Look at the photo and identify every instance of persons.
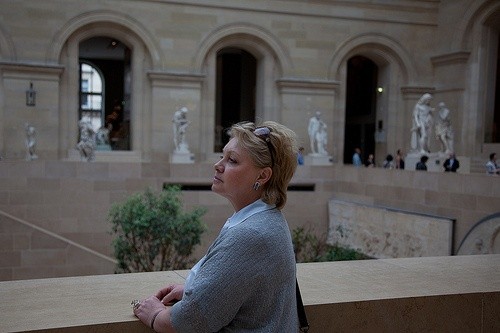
[296,143,500,180]
[1,88,460,173]
[133,119,302,333]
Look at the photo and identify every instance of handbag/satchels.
[296,277,309,331]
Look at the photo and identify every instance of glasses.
[253,127,276,170]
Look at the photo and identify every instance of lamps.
[378,86,383,95]
[25,82,36,106]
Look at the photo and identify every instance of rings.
[131,299,142,310]
